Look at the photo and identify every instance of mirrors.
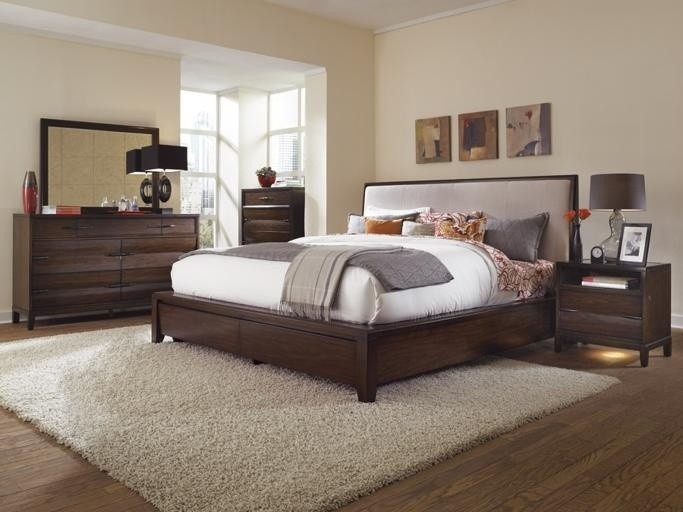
[39,116,158,213]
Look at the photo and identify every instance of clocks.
[591,246,603,262]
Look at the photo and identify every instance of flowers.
[564,206,589,223]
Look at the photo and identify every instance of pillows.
[344,205,551,264]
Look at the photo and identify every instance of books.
[41,204,82,215]
[581,276,640,291]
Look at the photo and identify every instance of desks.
[12,210,199,329]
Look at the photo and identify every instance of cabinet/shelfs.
[238,187,303,247]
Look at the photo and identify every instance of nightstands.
[554,257,673,366]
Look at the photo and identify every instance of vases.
[571,223,582,259]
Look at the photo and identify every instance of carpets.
[0,321,622,510]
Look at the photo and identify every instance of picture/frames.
[616,221,652,267]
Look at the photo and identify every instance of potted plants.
[255,165,276,188]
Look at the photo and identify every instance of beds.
[146,173,580,407]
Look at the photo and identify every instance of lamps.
[122,148,152,204]
[589,170,645,261]
[142,143,188,213]
